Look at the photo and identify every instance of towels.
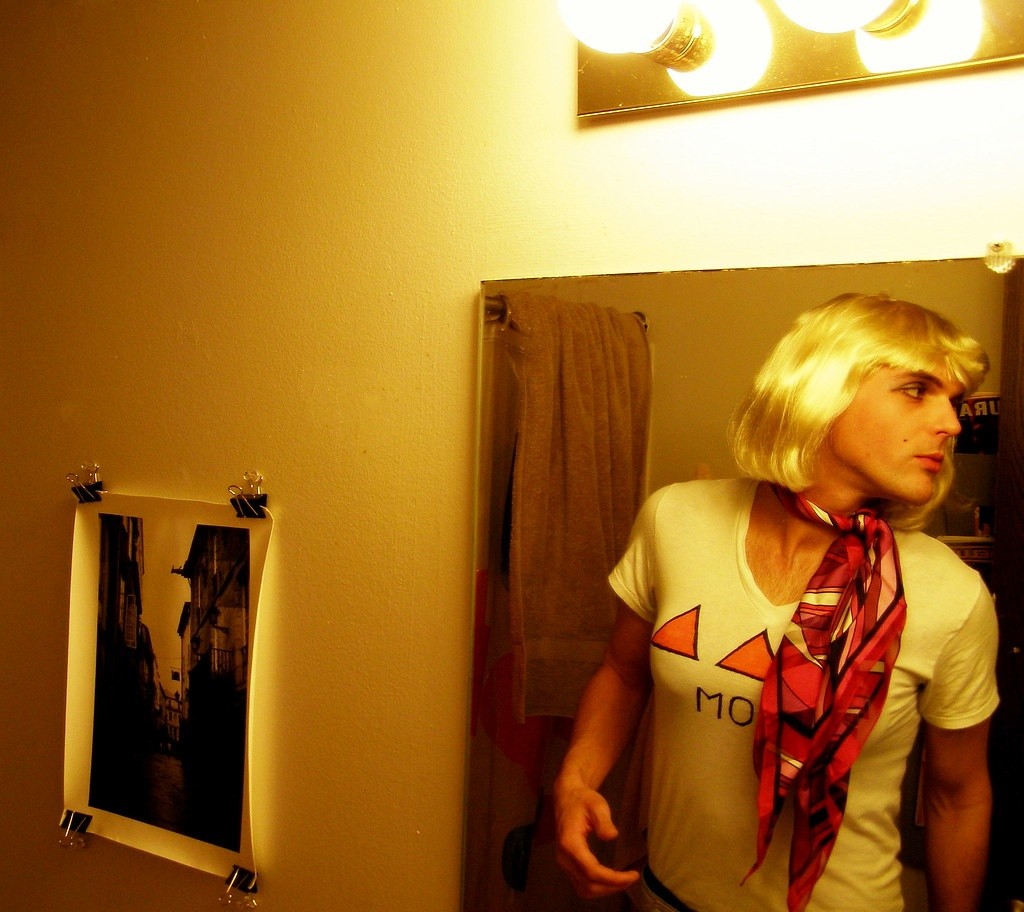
[486,289,652,726]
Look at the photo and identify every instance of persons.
[553,292,1000,912]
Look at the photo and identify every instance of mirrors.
[466,255,1024,912]
